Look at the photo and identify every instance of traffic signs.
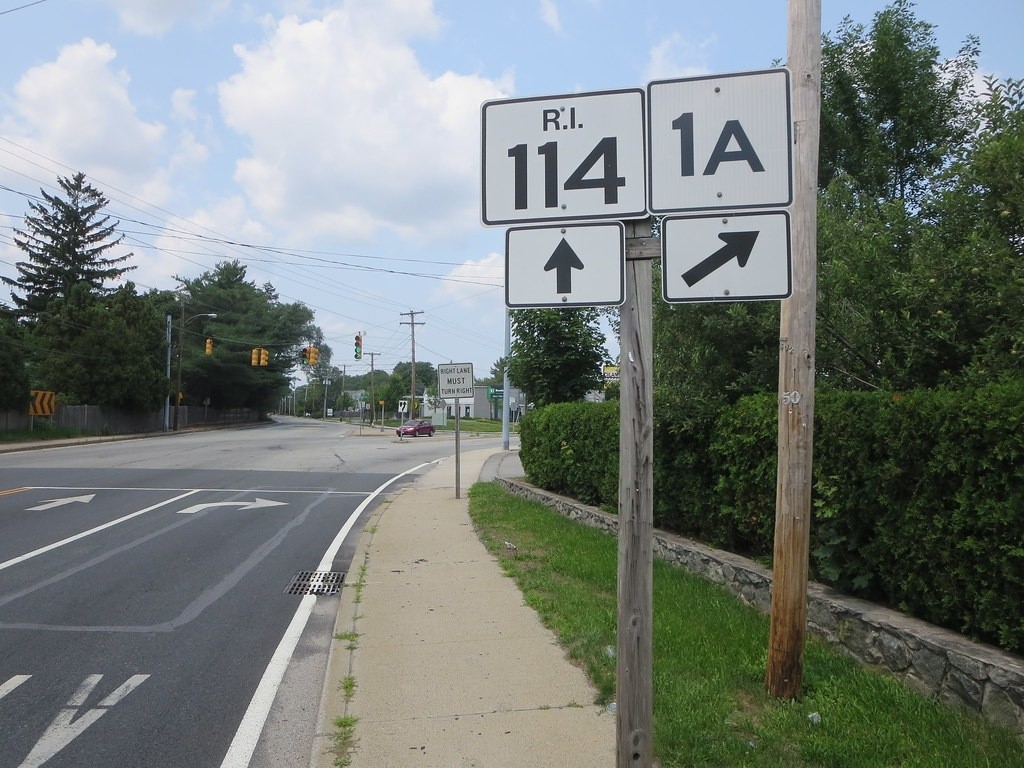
[30,390,54,415]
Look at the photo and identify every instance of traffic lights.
[260,349,268,366]
[355,335,362,359]
[310,347,318,364]
[302,348,310,365]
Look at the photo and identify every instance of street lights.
[173,314,217,431]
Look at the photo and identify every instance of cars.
[396,419,436,437]
